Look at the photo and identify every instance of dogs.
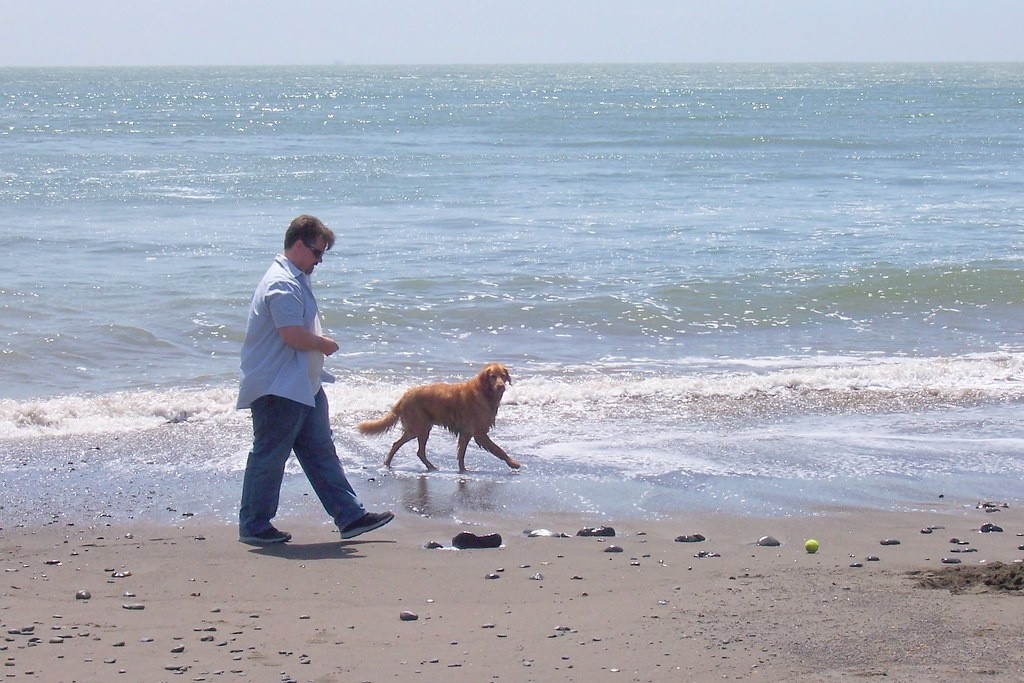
[358,363,520,471]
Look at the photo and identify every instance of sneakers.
[340,510,394,539]
[239,525,292,544]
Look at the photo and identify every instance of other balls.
[804,539,821,554]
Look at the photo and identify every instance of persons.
[235,215,395,544]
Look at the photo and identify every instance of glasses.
[308,245,323,258]
